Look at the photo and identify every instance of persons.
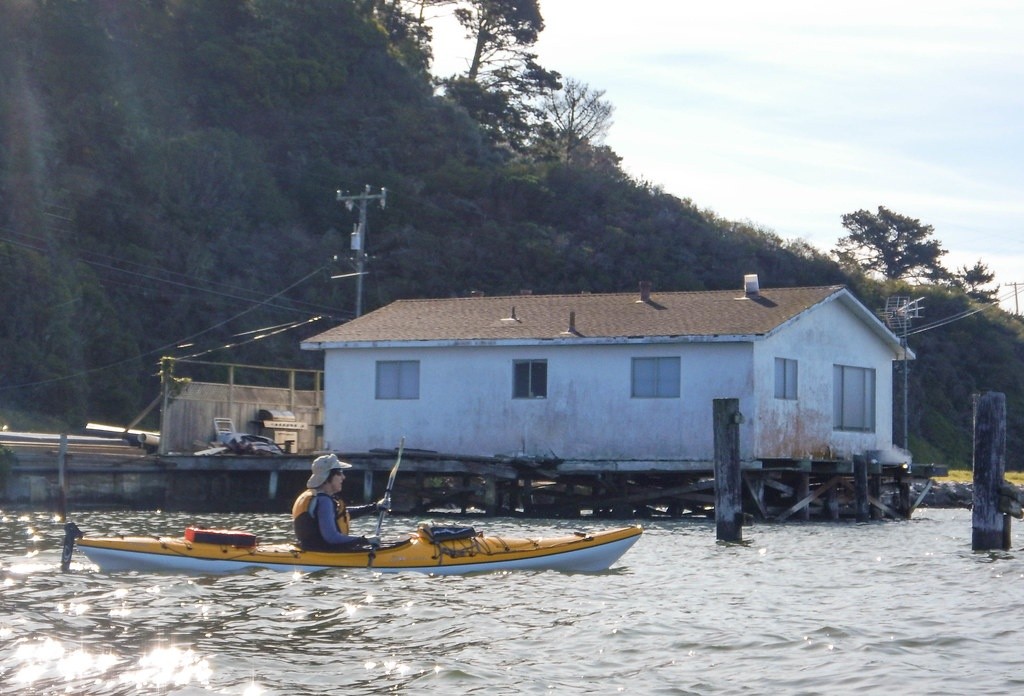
[292,453,392,551]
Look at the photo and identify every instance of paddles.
[366,433,405,570]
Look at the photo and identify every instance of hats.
[306,454,353,489]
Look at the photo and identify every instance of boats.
[215,430,284,456]
[60,521,645,578]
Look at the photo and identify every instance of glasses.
[331,470,343,476]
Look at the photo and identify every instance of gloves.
[366,536,381,547]
[376,499,392,515]
